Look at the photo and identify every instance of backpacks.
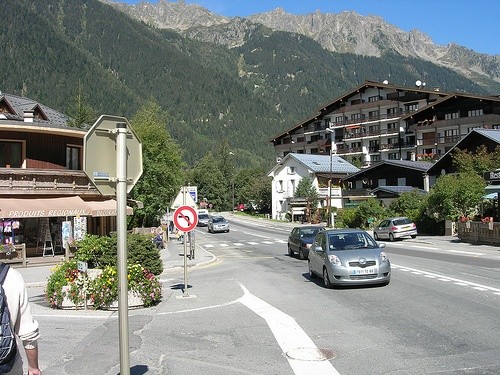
[0,262,18,373]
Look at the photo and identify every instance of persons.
[0,262,44,375]
[157,226,184,249]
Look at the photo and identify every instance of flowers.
[43,258,162,309]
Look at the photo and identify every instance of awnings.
[0,196,144,218]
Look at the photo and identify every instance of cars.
[197,213,211,227]
[373,216,419,241]
[308,227,391,289]
[288,226,330,260]
[207,215,229,233]
[237,199,263,212]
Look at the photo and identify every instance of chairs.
[334,240,344,247]
